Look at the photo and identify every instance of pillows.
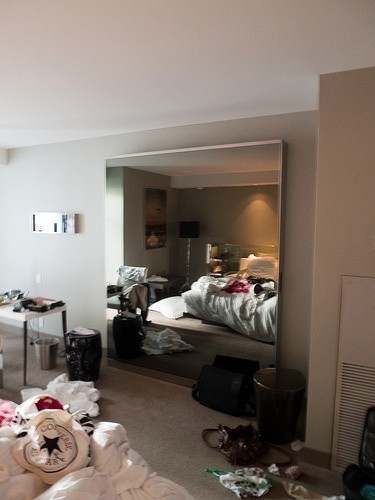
[246,255,274,271]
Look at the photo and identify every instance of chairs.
[107,266,148,316]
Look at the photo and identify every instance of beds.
[186,255,277,342]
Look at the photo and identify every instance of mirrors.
[105,139,289,390]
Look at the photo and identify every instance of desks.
[0,305,68,385]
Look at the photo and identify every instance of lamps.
[179,219,202,293]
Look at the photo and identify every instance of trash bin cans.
[34,337,59,371]
[252,367,307,446]
